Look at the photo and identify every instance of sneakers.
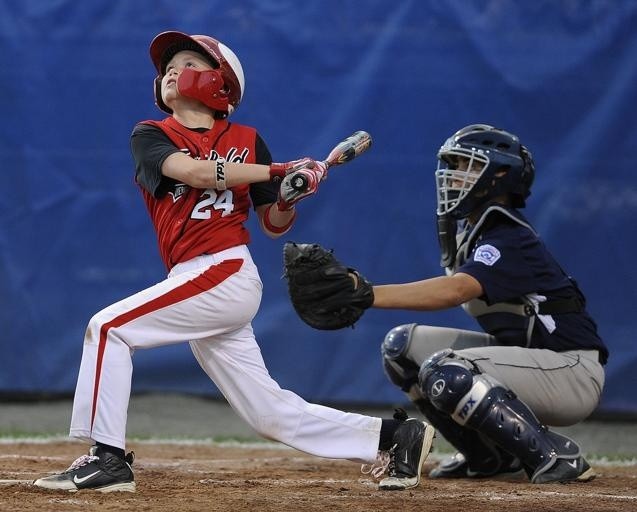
[378,419,435,490]
[34,446,136,496]
[535,455,596,483]
[430,451,525,480]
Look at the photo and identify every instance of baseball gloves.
[281,243,373,329]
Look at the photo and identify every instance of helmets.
[436,124,535,218]
[149,31,244,119]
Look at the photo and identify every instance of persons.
[31,28,436,492]
[283,122,611,486]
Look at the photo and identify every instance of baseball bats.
[291,131,371,190]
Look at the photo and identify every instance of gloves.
[272,158,327,211]
